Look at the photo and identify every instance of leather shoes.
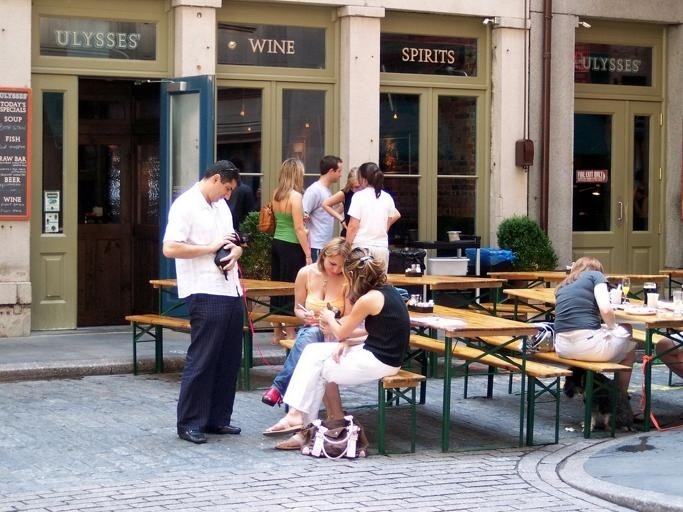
[261,387,284,407]
[179,429,207,444]
[199,424,241,434]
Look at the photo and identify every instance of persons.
[300,155,344,269]
[265,157,313,346]
[553,257,640,426]
[262,247,411,450]
[163,159,250,444]
[657,330,682,380]
[323,166,401,243]
[260,237,370,409]
[344,162,399,279]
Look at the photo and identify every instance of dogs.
[214,231,253,281]
[564,367,644,433]
[326,302,342,319]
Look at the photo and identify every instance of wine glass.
[622,278,631,304]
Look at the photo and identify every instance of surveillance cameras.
[582,21,591,29]
[483,18,489,25]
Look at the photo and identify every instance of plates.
[623,307,657,315]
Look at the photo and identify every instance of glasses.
[218,167,240,174]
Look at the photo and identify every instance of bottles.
[409,293,423,305]
[410,264,422,273]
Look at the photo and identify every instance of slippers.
[276,433,304,450]
[263,417,305,438]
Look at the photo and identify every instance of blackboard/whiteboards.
[0,87,32,220]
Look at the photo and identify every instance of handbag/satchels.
[519,320,557,353]
[258,202,275,234]
[301,415,369,460]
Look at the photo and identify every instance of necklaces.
[321,279,328,290]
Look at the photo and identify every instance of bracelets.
[306,256,311,259]
[341,218,347,223]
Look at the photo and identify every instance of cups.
[673,291,683,313]
[643,282,656,307]
[611,289,622,304]
[647,293,659,307]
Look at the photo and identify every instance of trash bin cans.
[466,248,515,303]
[389,249,426,302]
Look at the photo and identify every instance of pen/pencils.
[298,304,315,320]
